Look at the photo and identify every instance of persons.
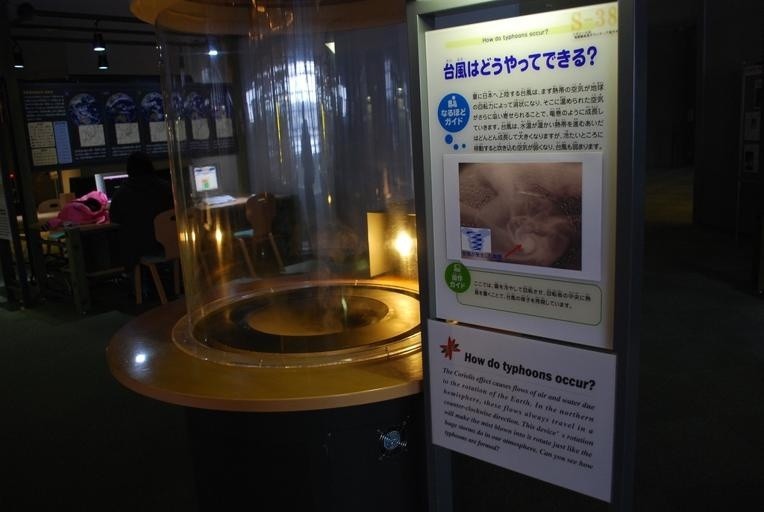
[109,150,173,297]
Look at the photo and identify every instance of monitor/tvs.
[103,174,128,204]
[188,162,224,198]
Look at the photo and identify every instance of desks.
[29,219,137,315]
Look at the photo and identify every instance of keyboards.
[201,194,236,205]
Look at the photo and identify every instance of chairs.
[134,192,286,306]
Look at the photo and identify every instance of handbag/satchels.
[48,190,108,228]
